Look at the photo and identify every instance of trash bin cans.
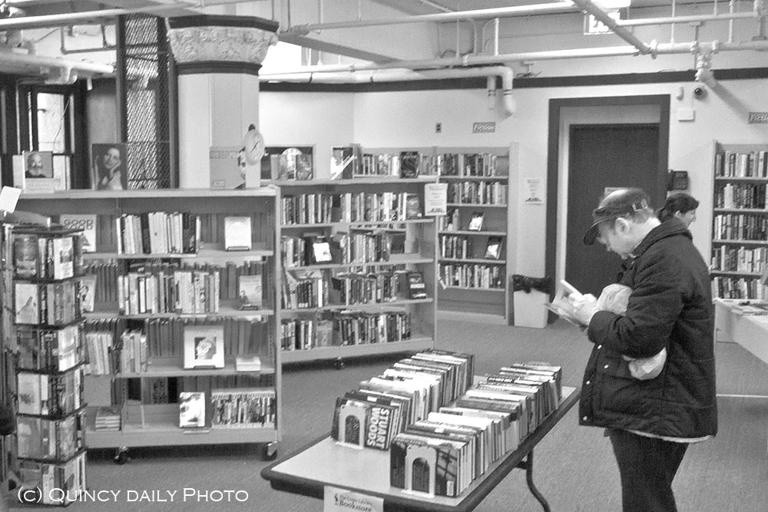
[513,275,552,329]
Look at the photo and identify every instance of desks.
[259,375,584,511]
[712,295,768,364]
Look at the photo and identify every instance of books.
[269,148,508,348]
[3,197,271,505]
[544,281,589,329]
[333,350,560,502]
[21,151,56,193]
[713,149,767,305]
[91,143,125,191]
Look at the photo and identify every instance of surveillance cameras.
[694,87,705,97]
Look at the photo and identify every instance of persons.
[25,153,53,175]
[98,146,121,187]
[572,186,719,512]
[660,193,700,227]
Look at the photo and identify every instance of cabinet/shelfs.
[2,187,288,451]
[349,143,523,325]
[712,135,767,306]
[254,172,446,366]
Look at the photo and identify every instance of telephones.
[665,170,687,190]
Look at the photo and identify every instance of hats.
[583,188,648,245]
